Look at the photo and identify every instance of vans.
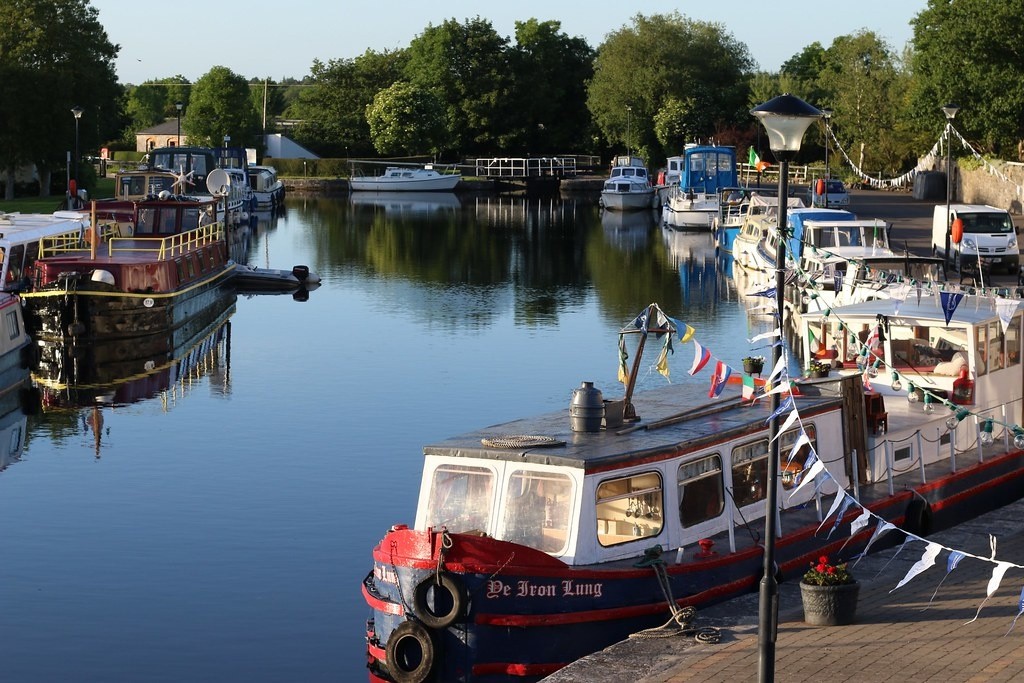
[932,203,1020,274]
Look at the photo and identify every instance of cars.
[805,177,852,211]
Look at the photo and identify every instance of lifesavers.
[412,570,462,629]
[27,346,40,370]
[385,620,434,683]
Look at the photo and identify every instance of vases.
[743,364,762,373]
[799,579,861,626]
[810,369,829,378]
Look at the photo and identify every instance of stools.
[874,411,888,435]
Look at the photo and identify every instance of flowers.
[809,360,832,372]
[741,355,767,366]
[804,554,856,587]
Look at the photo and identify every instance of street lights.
[71,105,85,184]
[625,101,633,157]
[175,101,184,147]
[750,91,835,683]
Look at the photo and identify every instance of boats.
[0,208,90,398]
[0,356,36,473]
[114,142,281,235]
[358,294,1024,683]
[600,162,655,211]
[602,205,656,253]
[18,278,239,459]
[350,166,462,192]
[351,189,460,213]
[711,182,944,336]
[821,107,835,208]
[655,140,738,228]
[18,168,241,346]
[941,103,960,282]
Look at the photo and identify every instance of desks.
[865,391,881,429]
